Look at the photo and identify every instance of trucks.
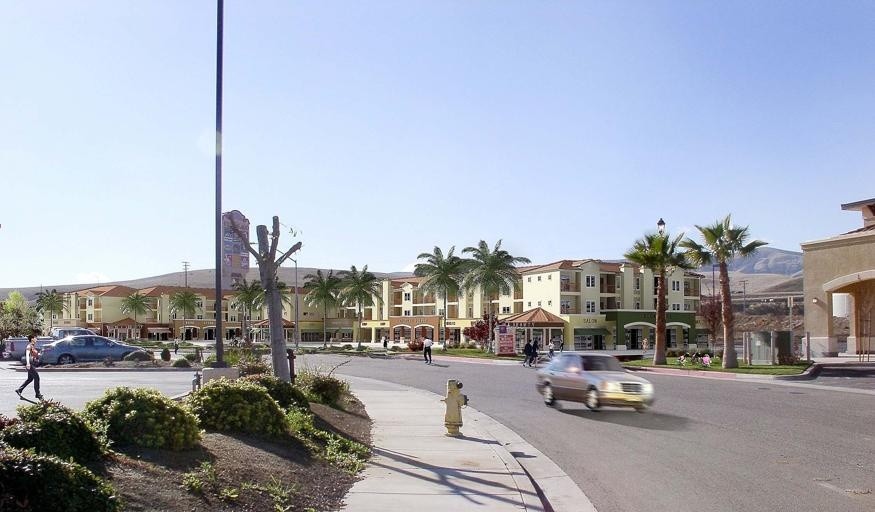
[1,327,100,360]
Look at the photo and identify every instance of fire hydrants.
[441,379,470,438]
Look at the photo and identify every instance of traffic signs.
[248,241,299,352]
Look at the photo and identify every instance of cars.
[37,334,147,364]
[532,350,655,414]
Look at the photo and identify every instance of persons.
[13,333,43,398]
[677,352,713,369]
[522,339,532,367]
[641,337,649,353]
[559,339,565,352]
[528,339,540,368]
[422,336,434,366]
[548,339,554,360]
[174,336,179,355]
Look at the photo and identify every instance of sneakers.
[15,390,43,399]
